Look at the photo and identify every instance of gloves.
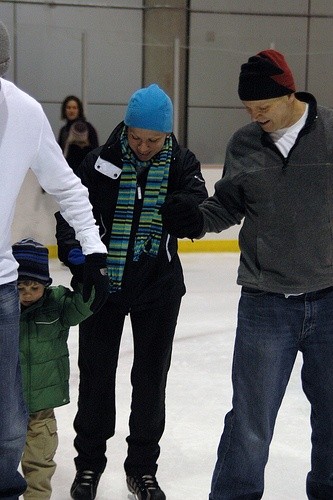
[157,193,204,238]
[66,246,109,314]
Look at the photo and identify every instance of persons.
[162,49,333,500]
[56,95,99,153]
[42,122,93,172]
[53,84,208,500]
[0,24,108,500]
[11,238,94,500]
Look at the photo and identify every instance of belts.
[242,285,333,302]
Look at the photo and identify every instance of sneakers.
[71,469,101,500]
[127,470,166,500]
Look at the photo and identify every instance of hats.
[238,50,296,101]
[124,83,173,133]
[12,238,52,287]
[0,21,10,77]
[67,122,90,149]
